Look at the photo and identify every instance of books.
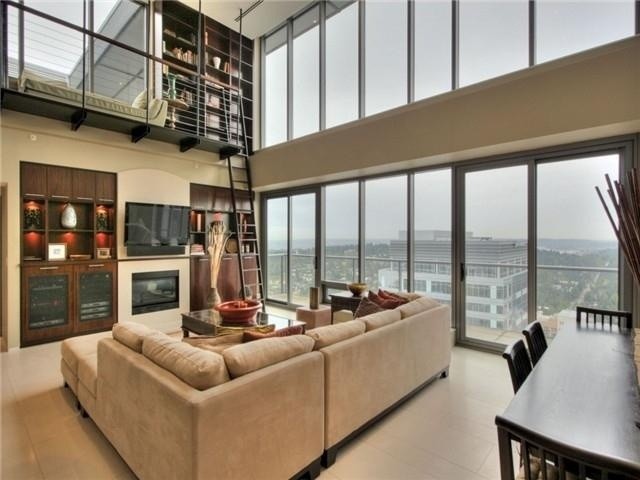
[163,27,244,146]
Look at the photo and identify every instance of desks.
[502,320,640,480]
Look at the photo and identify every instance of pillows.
[352,287,421,319]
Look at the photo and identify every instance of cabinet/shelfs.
[188,181,258,312]
[19,161,118,348]
[156,0,255,154]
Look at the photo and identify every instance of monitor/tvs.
[124,201,192,247]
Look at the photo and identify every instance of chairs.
[495,306,632,480]
